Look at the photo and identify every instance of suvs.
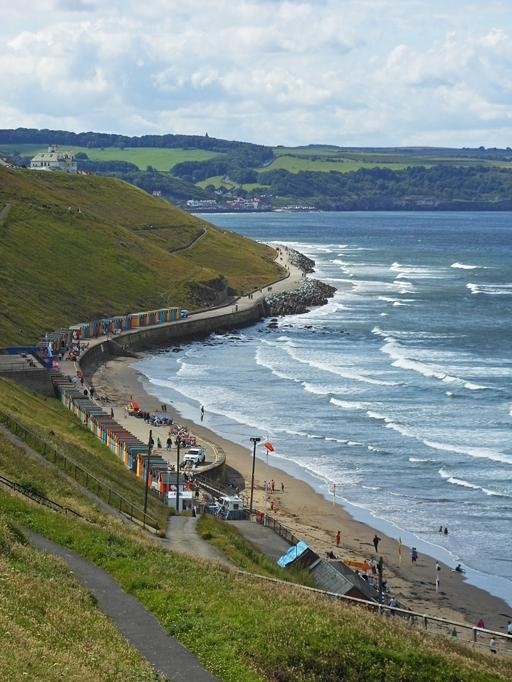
[184,448,205,464]
[209,503,219,511]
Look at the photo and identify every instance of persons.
[372,534,381,552]
[335,529,342,548]
[435,576,440,592]
[108,394,195,450]
[200,405,205,422]
[444,527,449,534]
[166,460,286,518]
[506,620,512,641]
[455,564,466,574]
[489,637,497,654]
[50,327,112,400]
[476,618,486,636]
[439,525,443,532]
[434,560,442,576]
[323,546,419,618]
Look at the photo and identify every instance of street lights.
[247,437,260,519]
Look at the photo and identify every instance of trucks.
[215,497,244,513]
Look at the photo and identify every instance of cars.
[180,309,189,317]
